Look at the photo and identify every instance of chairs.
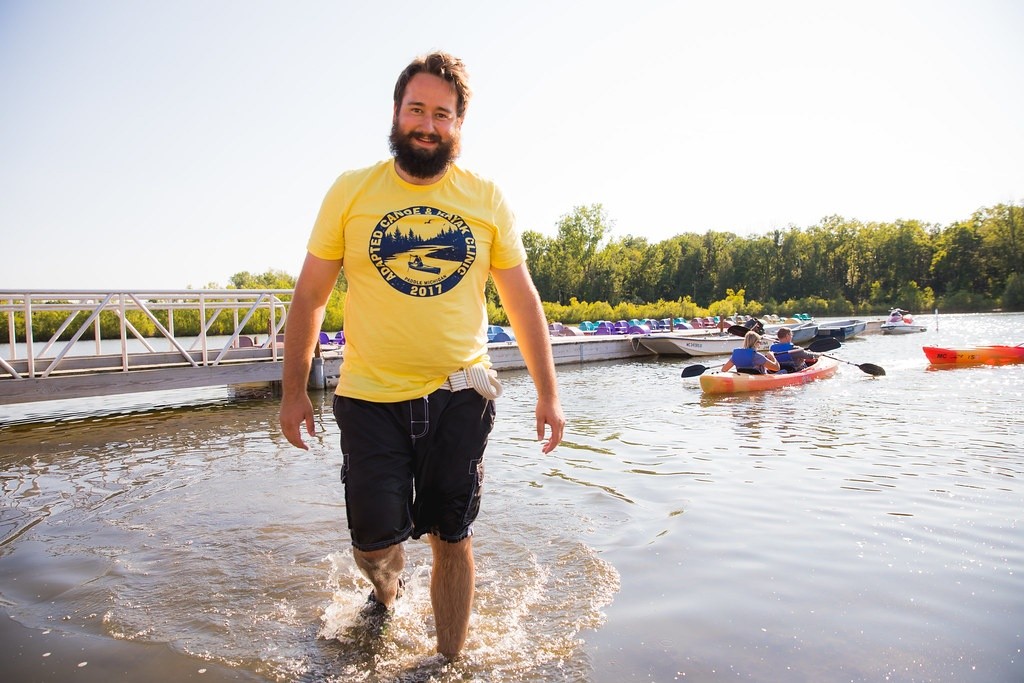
[737,366,762,375]
[766,365,797,375]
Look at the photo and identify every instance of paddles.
[727,325,886,376]
[680,337,841,377]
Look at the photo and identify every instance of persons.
[887,309,903,322]
[279,49,565,658]
[722,330,787,375]
[771,327,823,370]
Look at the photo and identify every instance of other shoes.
[367,580,407,620]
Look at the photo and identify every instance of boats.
[880,308,928,335]
[921,342,1024,371]
[816,319,868,343]
[699,355,841,394]
[631,321,819,358]
